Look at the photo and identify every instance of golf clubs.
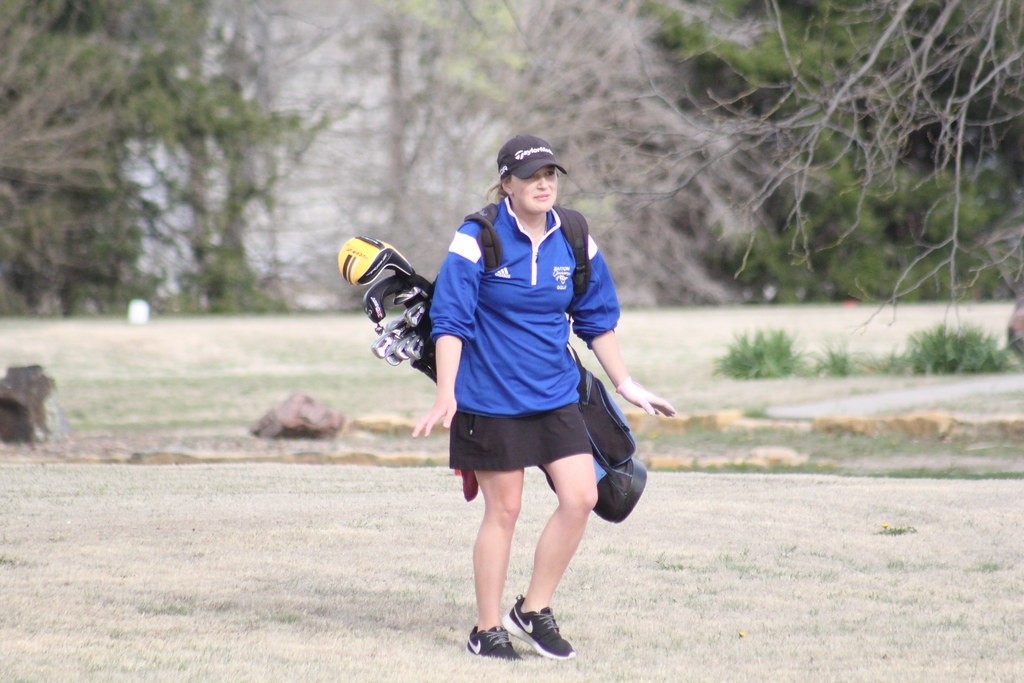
[336,234,429,369]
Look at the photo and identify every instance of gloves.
[617,377,676,418]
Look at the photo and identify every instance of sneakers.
[502,594,575,660]
[466,624,524,661]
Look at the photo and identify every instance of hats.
[497,135,567,178]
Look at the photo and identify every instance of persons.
[412,135,675,661]
